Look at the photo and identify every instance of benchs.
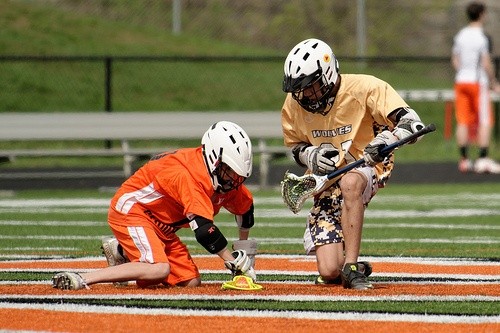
[0,111,292,190]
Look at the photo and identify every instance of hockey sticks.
[280,121,436,214]
[221,270,262,290]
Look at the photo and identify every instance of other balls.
[235,278,249,288]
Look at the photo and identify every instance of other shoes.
[342,260,374,290]
[458,159,472,171]
[316,273,341,284]
[474,158,500,174]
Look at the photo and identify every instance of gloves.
[232,239,257,267]
[224,249,257,284]
[308,146,339,176]
[362,130,396,166]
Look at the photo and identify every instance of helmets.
[200,121,253,194]
[282,37,340,114]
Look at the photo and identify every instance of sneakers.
[100,236,129,287]
[51,272,86,290]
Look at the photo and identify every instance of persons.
[281,38,425,290]
[450,3,500,175]
[51,121,256,290]
